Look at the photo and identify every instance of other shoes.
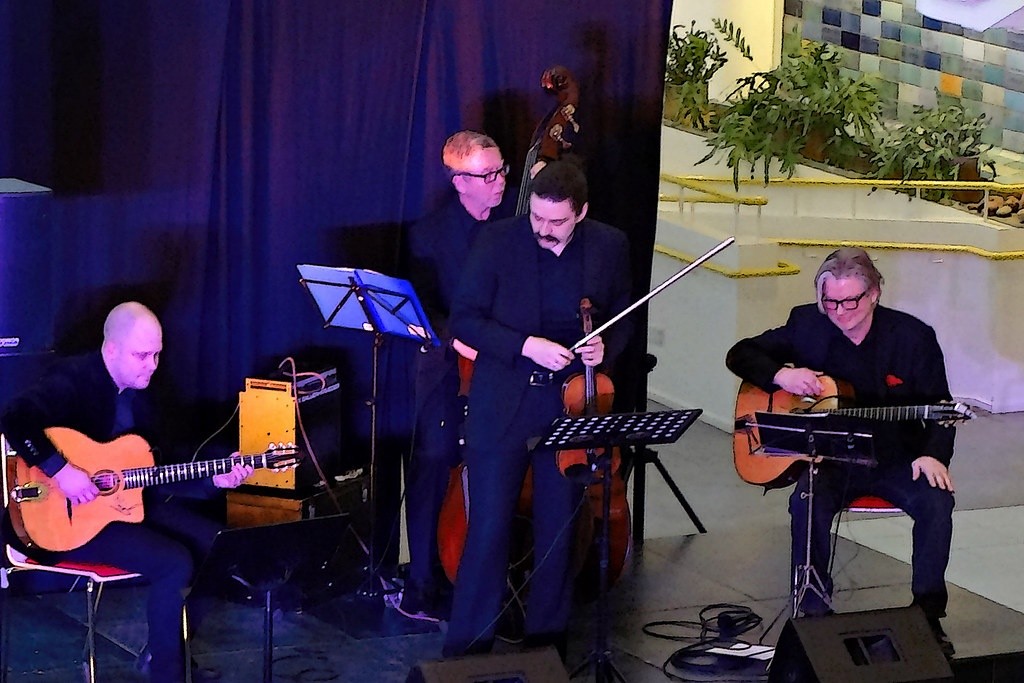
[133,643,221,683]
[934,622,955,656]
[398,586,450,622]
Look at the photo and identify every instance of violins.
[553,295,624,487]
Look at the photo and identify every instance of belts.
[528,369,579,386]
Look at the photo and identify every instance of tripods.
[526,409,704,683]
[627,355,709,548]
[755,411,877,675]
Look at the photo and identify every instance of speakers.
[767,605,954,683]
[405,644,575,683]
[239,364,343,499]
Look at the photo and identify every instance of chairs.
[834,496,910,524]
[0,433,192,683]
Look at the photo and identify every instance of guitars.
[732,369,979,490]
[4,424,308,555]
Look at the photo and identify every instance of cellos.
[428,60,635,603]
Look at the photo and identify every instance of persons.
[726,247,958,660]
[1,300,255,683]
[394,129,530,644]
[440,160,632,661]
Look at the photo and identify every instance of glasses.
[821,288,869,311]
[453,163,510,184]
